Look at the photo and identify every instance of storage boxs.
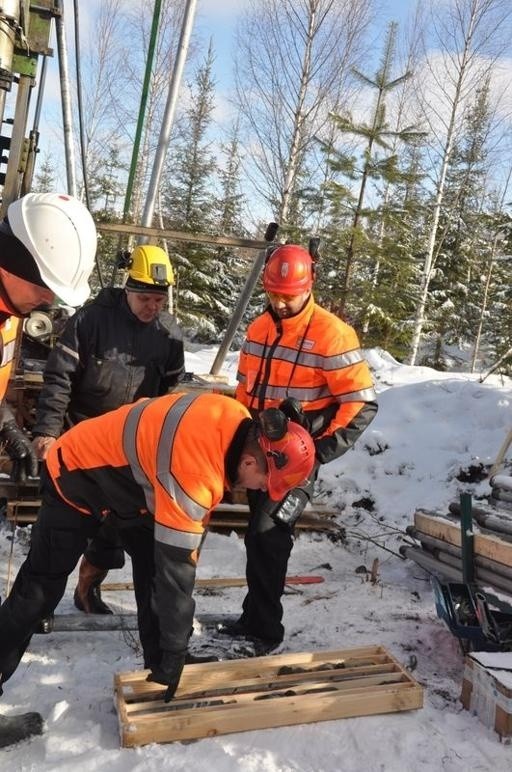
[459,650,512,737]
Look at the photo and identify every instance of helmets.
[7,191,98,311]
[263,243,312,297]
[122,244,173,290]
[257,418,317,502]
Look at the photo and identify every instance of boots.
[71,556,113,615]
[37,614,54,632]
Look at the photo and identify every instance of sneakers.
[226,634,283,661]
[217,615,256,634]
[0,710,46,749]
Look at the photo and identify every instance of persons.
[1,190,99,481]
[209,243,381,642]
[31,246,187,634]
[0,391,317,706]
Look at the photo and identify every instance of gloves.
[2,424,38,483]
[147,652,183,703]
